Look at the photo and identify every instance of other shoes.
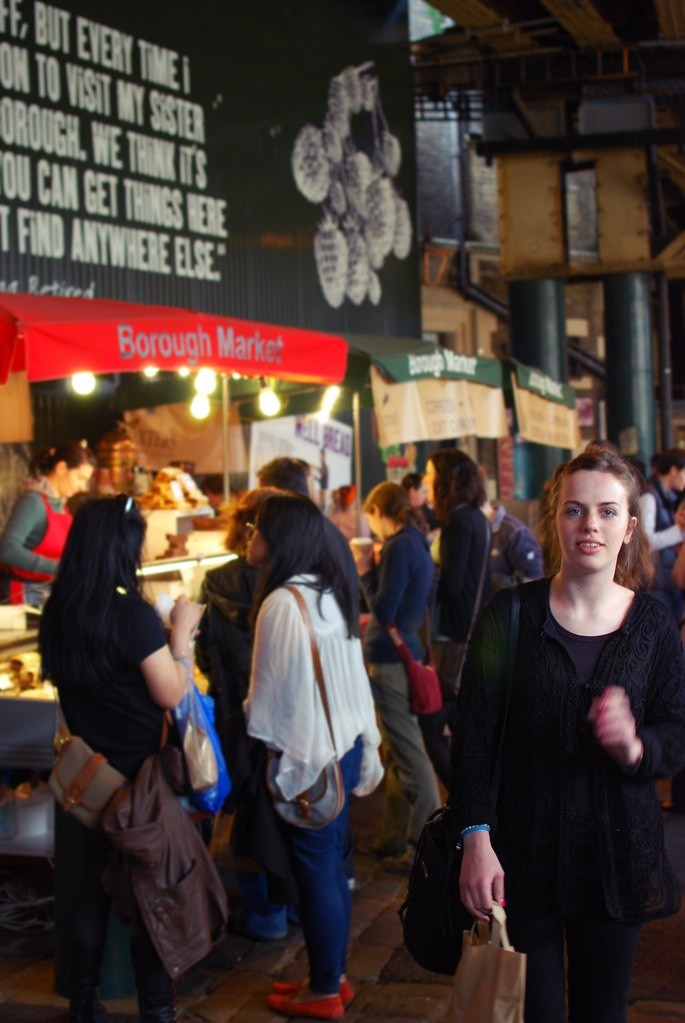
[228,892,250,933]
[69,990,108,1023]
[380,850,412,876]
[355,831,395,855]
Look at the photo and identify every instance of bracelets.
[460,823,490,837]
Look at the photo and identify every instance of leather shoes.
[266,992,345,1021]
[267,977,352,1006]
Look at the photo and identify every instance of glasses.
[114,493,134,515]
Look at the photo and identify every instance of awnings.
[0,291,348,504]
[501,358,583,449]
[333,333,509,448]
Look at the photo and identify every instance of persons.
[585,439,685,593]
[361,480,443,874]
[401,449,546,797]
[447,442,685,1023]
[0,424,385,1022]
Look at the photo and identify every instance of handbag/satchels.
[431,636,466,698]
[395,805,483,973]
[174,658,232,816]
[449,902,526,1023]
[50,734,124,829]
[408,655,441,716]
[267,760,346,832]
